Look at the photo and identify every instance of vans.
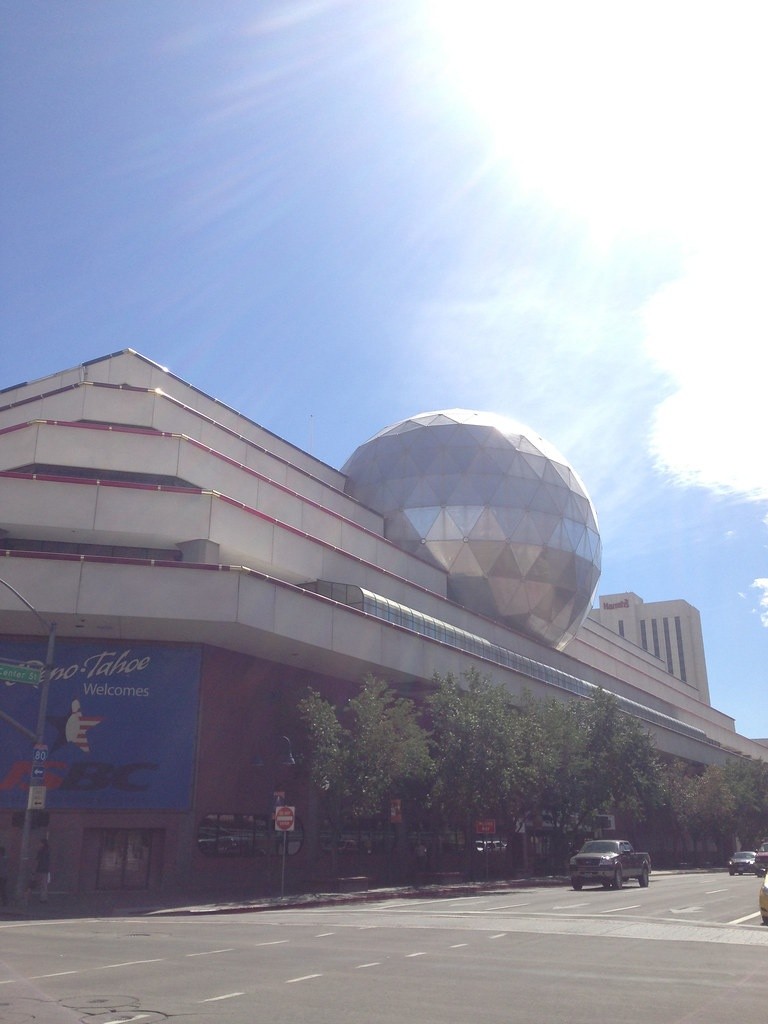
[755,841,768,878]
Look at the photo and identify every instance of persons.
[413,841,427,867]
[35,839,50,903]
[1,847,8,906]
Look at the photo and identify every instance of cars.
[197,817,240,859]
[729,851,756,876]
[473,840,508,856]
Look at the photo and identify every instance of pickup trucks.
[569,839,652,891]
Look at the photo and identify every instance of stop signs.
[276,807,294,830]
